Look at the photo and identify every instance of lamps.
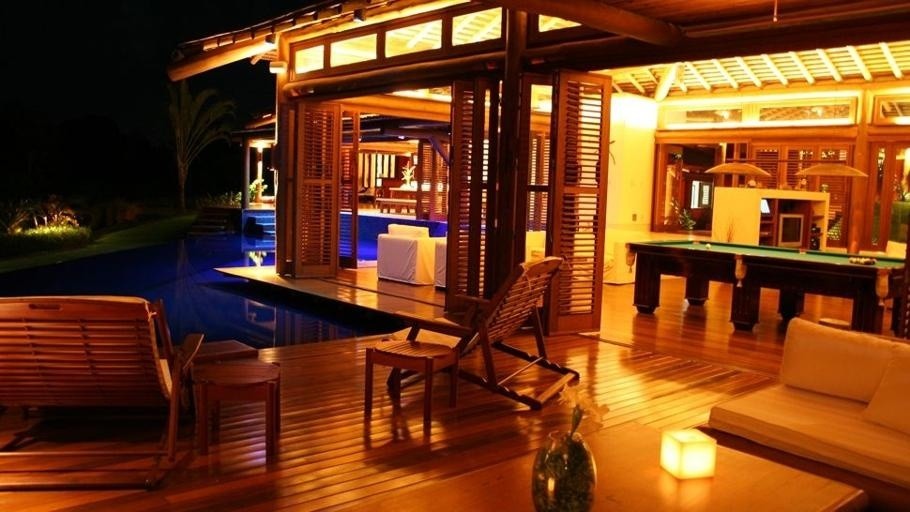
[660,428,717,481]
[269,62,287,73]
[266,24,276,44]
[353,7,366,21]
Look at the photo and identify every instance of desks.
[389,188,448,213]
[629,239,907,336]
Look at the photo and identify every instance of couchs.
[709,317,909,512]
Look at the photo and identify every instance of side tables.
[365,340,459,420]
[189,358,281,462]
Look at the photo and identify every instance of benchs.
[376,197,432,215]
[159,339,259,364]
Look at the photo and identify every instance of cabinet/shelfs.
[711,187,830,252]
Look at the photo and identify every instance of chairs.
[434,241,447,291]
[1,295,205,491]
[382,256,580,411]
[377,233,447,285]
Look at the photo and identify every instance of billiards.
[849,256,877,265]
[705,243,711,249]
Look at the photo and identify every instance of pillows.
[388,224,430,238]
[861,343,910,433]
